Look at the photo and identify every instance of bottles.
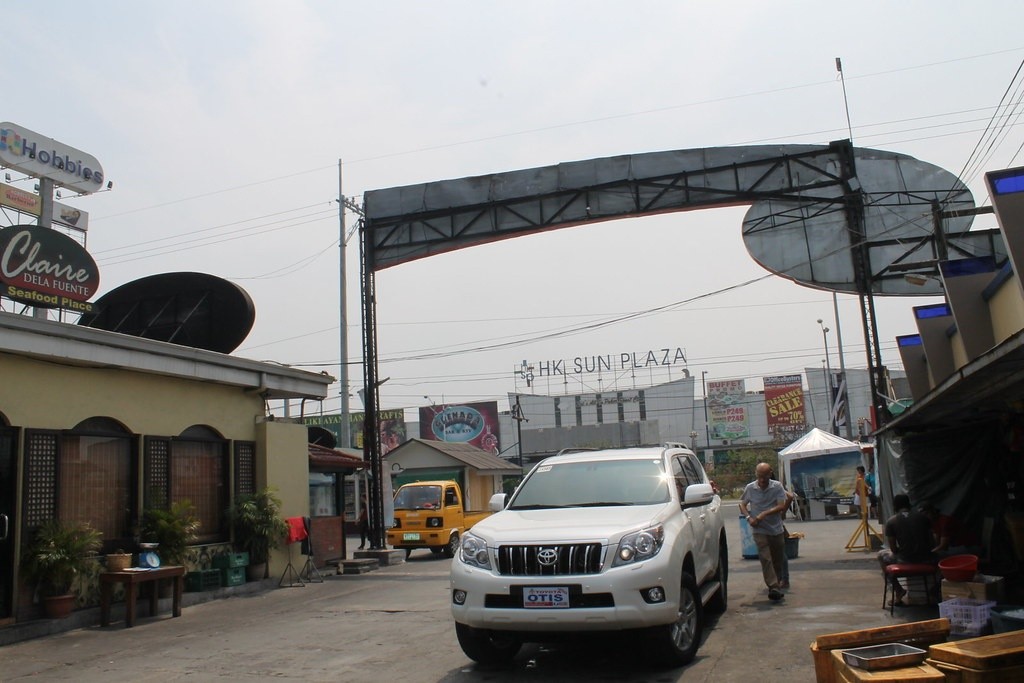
[193,550,243,572]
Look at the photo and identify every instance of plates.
[842,643,927,668]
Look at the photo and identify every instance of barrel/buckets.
[937,555,979,582]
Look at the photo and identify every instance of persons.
[738,463,794,602]
[854,473,866,517]
[481,433,499,456]
[381,420,399,456]
[353,502,373,547]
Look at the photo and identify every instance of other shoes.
[888,590,907,605]
[768,588,784,601]
[780,583,789,588]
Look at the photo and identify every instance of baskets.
[937,596,997,637]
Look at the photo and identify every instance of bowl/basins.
[987,605,1024,634]
[139,543,159,548]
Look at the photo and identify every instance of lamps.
[905,273,944,289]
[1,152,113,199]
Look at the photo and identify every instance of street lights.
[423,394,437,442]
[817,318,840,436]
[702,370,710,449]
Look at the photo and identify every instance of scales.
[139,543,160,569]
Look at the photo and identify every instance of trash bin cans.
[739,511,760,559]
[785,538,799,558]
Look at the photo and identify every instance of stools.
[883,563,936,617]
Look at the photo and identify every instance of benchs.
[938,516,994,572]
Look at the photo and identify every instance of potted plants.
[22,517,104,619]
[132,495,202,598]
[225,482,291,582]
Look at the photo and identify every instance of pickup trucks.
[385,479,500,559]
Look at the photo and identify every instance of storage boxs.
[941,575,1005,605]
[938,597,997,637]
[810,619,1024,683]
[221,566,247,586]
[896,575,937,605]
[184,568,222,592]
[211,550,249,569]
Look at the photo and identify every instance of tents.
[777,426,870,520]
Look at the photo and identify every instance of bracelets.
[745,515,750,522]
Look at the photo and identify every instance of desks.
[99,562,185,628]
[807,497,856,520]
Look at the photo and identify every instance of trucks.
[449,440,730,669]
